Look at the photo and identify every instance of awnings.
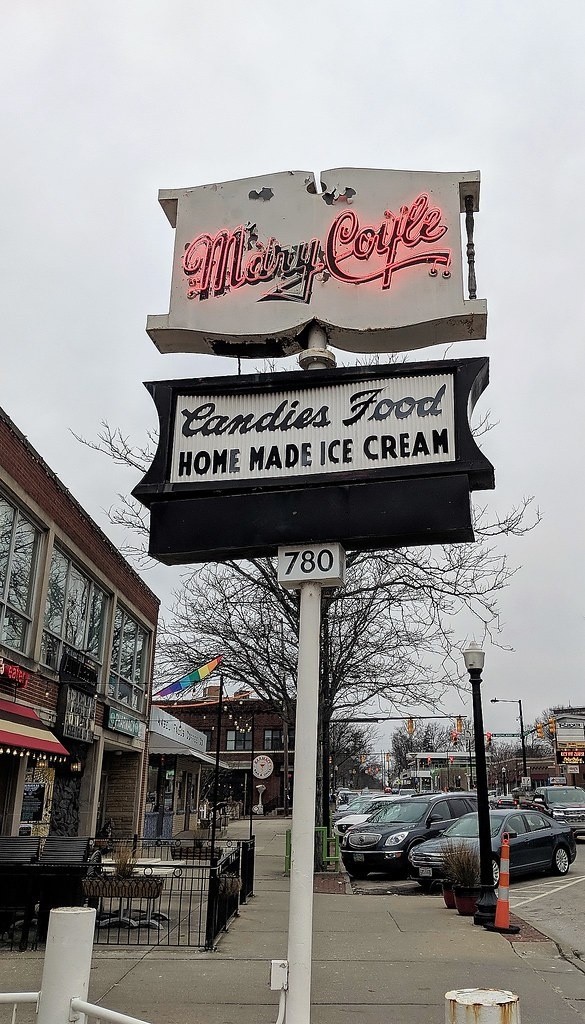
[0,698,70,762]
[150,731,231,769]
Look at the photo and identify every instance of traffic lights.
[536,723,543,739]
[450,756,453,764]
[369,768,370,772]
[427,757,431,766]
[548,718,555,733]
[453,732,457,745]
[487,731,491,746]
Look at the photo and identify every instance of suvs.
[533,787,585,838]
[333,792,412,843]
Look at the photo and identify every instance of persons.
[444,786,450,792]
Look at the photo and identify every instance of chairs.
[530,817,541,831]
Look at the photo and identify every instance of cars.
[407,807,577,888]
[341,791,478,877]
[147,792,180,810]
[488,787,534,810]
[332,787,416,825]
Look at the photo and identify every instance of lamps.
[69,754,82,776]
[89,692,98,700]
[45,683,49,698]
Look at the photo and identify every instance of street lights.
[501,766,507,795]
[458,776,461,787]
[490,699,528,778]
[463,639,499,925]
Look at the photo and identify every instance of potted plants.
[431,836,480,915]
[82,841,164,899]
[170,830,224,860]
